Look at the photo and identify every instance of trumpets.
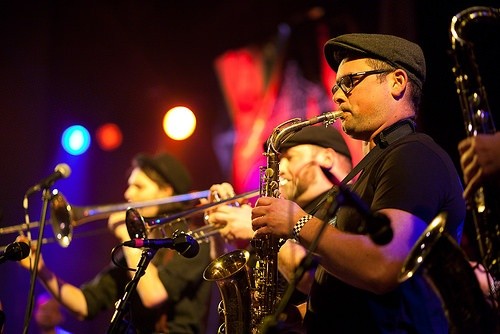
[124,179,286,244]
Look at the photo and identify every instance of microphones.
[317,165,394,246]
[26,163,72,196]
[0,242,30,265]
[124,237,177,250]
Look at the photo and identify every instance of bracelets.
[292,214,316,242]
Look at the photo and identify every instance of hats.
[262,124,352,158]
[132,151,192,205]
[323,33,426,92]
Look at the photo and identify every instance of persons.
[459,132,500,200]
[251,34,467,334]
[203,125,353,334]
[14,154,212,334]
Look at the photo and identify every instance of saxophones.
[202,107,345,334]
[395,5,500,334]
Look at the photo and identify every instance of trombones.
[0,187,209,256]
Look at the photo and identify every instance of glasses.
[330,69,396,95]
